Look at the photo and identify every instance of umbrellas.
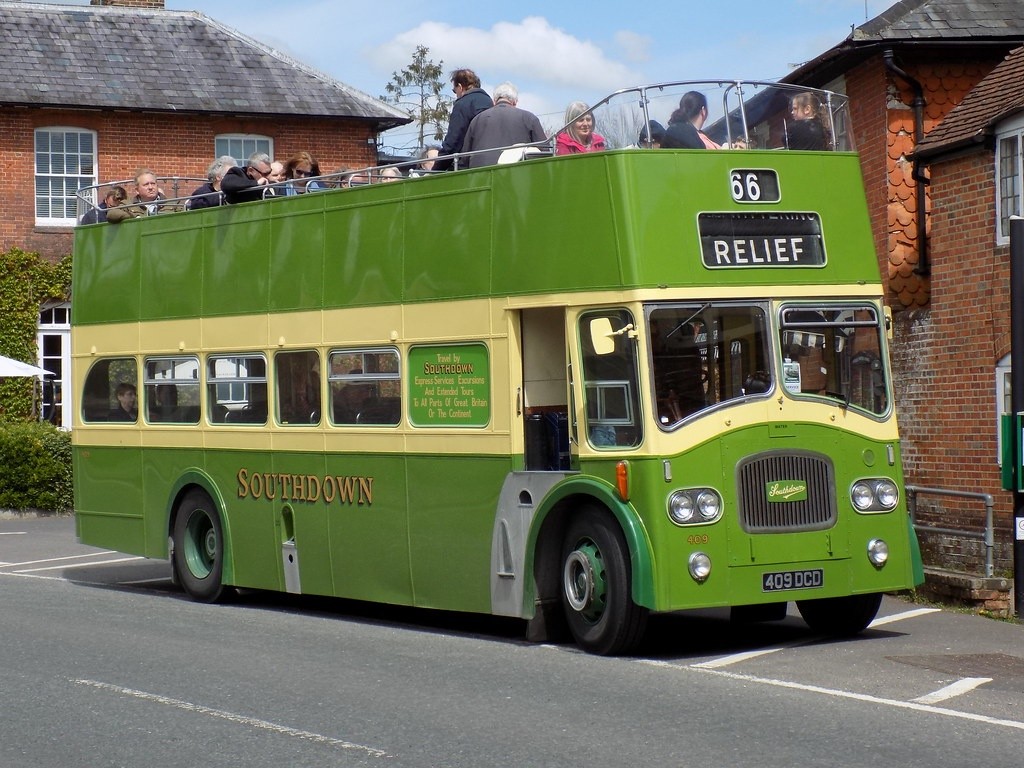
[0,354,56,377]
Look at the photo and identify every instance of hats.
[639,120,666,142]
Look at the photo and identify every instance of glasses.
[294,167,313,176]
[252,166,272,176]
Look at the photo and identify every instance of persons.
[79,69,829,223]
[665,323,705,421]
[104,369,374,425]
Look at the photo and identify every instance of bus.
[66,78,925,661]
